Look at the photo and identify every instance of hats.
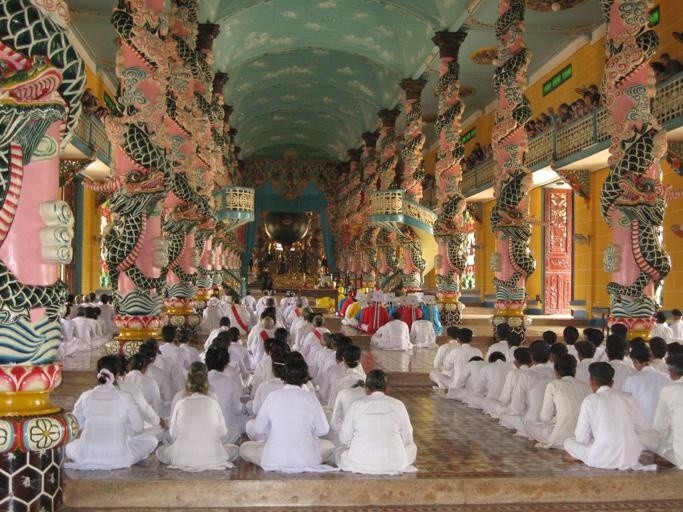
[355,289,435,305]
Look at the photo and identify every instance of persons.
[559,359,658,473]
[60,356,159,471]
[643,355,683,471]
[240,357,338,474]
[335,366,424,474]
[429,303,680,451]
[153,368,241,471]
